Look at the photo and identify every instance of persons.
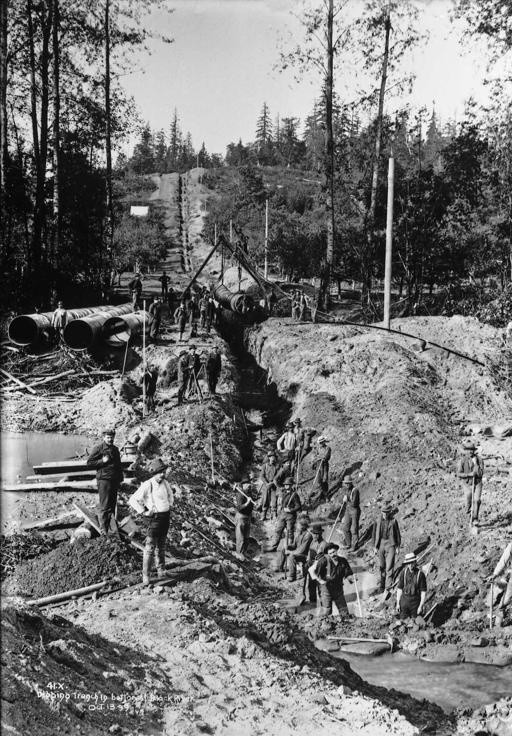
[87,271,482,620]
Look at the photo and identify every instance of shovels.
[206,434,218,489]
[469,477,479,535]
[298,567,310,606]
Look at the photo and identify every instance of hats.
[241,477,249,484]
[148,459,167,473]
[324,543,339,554]
[342,475,354,484]
[317,436,326,443]
[267,452,278,457]
[382,504,392,514]
[283,478,292,485]
[310,524,324,535]
[180,344,218,356]
[103,429,115,435]
[286,417,302,429]
[401,552,416,564]
[462,442,478,451]
[281,457,289,463]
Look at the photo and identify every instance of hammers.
[233,483,258,506]
